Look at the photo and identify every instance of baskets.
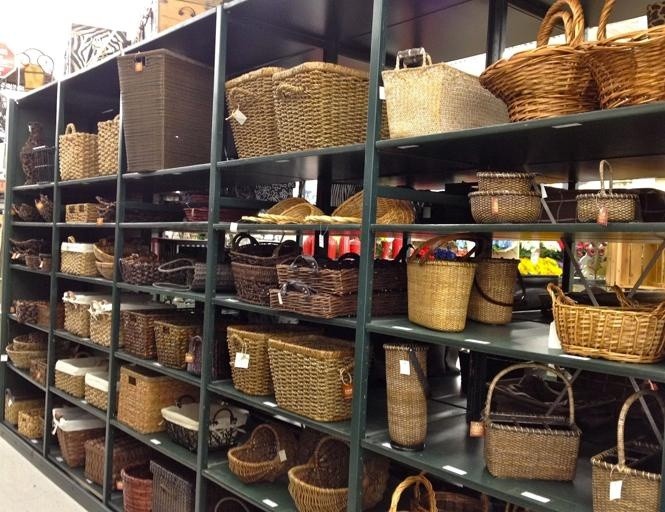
[121,462,152,512]
[92,239,133,263]
[59,235,98,276]
[97,114,120,175]
[467,190,542,224]
[15,299,49,325]
[149,458,195,512]
[83,436,140,491]
[240,197,310,224]
[6,344,65,369]
[271,62,391,154]
[546,281,665,364]
[88,300,124,349]
[381,47,509,139]
[233,279,277,306]
[62,290,90,338]
[286,436,390,512]
[25,255,39,267]
[58,122,97,180]
[185,335,224,378]
[579,0,665,110]
[480,363,583,482]
[122,309,179,360]
[258,203,325,226]
[160,394,250,454]
[54,357,108,398]
[181,204,239,224]
[227,424,289,485]
[387,470,529,512]
[275,255,405,296]
[118,255,167,285]
[34,197,54,222]
[31,147,54,183]
[116,47,212,173]
[226,323,273,397]
[36,303,64,329]
[230,260,275,283]
[589,389,664,512]
[476,171,536,192]
[94,259,114,280]
[479,0,598,123]
[39,254,51,271]
[12,202,41,222]
[9,236,45,263]
[84,371,119,413]
[16,407,44,439]
[29,358,47,386]
[224,68,285,158]
[13,333,47,351]
[304,189,415,224]
[229,232,300,267]
[576,160,638,224]
[51,407,106,467]
[267,333,354,422]
[4,387,45,425]
[116,364,200,435]
[65,202,98,223]
[154,319,202,369]
[268,281,406,320]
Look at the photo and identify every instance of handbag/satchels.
[384,341,430,447]
[455,240,526,324]
[406,257,478,333]
[152,257,233,292]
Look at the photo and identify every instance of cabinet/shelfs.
[0,1,665,512]
